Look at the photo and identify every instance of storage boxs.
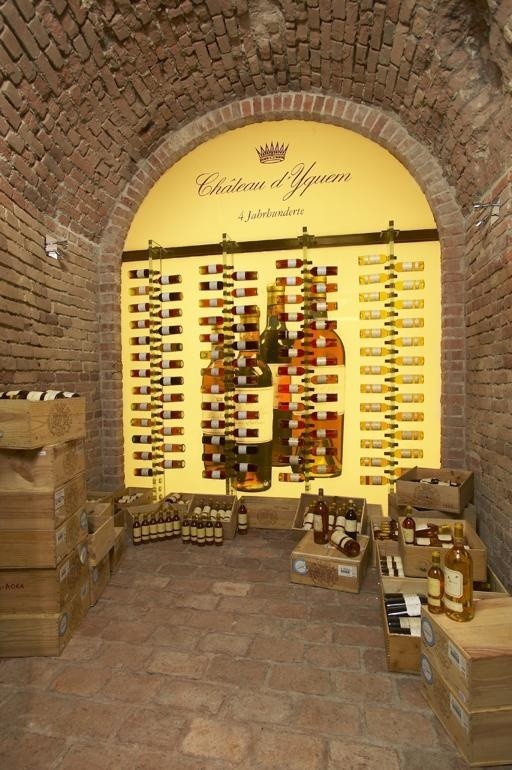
[0,442,89,661]
[0,391,90,448]
[287,466,511,770]
[89,484,240,607]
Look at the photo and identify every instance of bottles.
[301,488,362,558]
[380,554,404,578]
[260,269,347,479]
[129,269,165,477]
[371,517,399,540]
[298,266,339,474]
[383,590,427,638]
[115,492,143,505]
[197,264,238,480]
[273,259,315,484]
[151,273,186,469]
[223,271,260,473]
[444,522,474,622]
[357,255,398,487]
[236,494,248,537]
[402,506,471,548]
[132,492,232,547]
[201,305,273,493]
[384,261,425,476]
[407,478,462,488]
[0,389,82,401]
[427,551,444,614]
